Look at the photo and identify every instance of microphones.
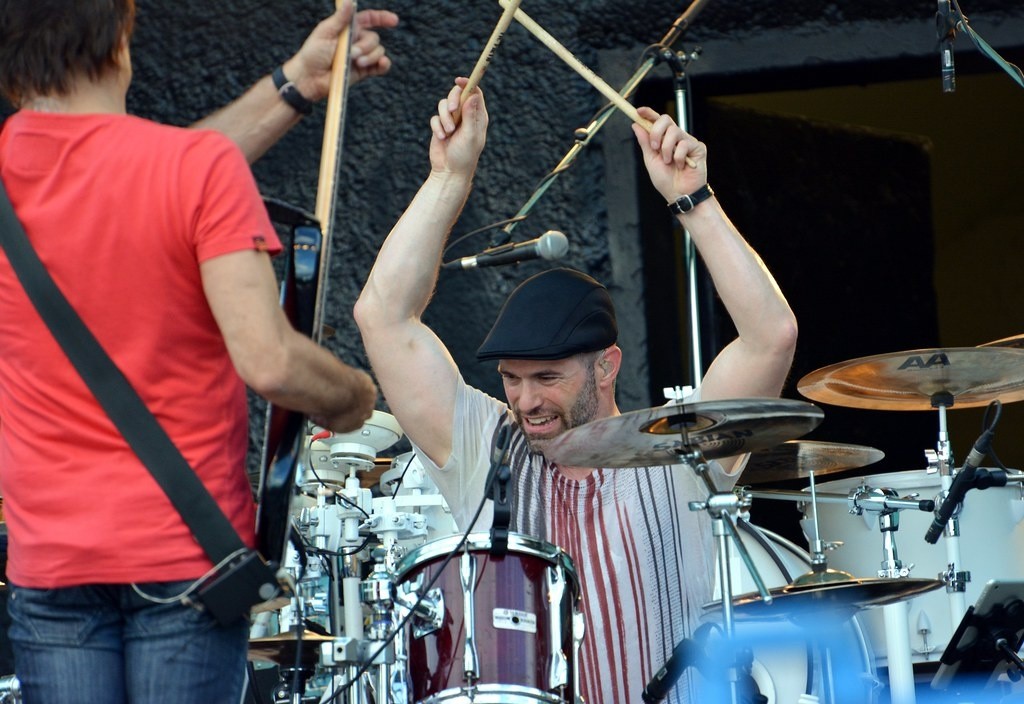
[642,620,717,704]
[445,229,569,272]
[926,430,993,545]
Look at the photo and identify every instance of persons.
[353,78,799,704]
[0,0,401,704]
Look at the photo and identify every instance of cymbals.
[704,442,885,482]
[342,458,394,489]
[697,568,948,633]
[976,334,1024,348]
[798,348,1023,411]
[248,628,338,671]
[538,397,824,466]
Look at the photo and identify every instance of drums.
[393,527,586,704]
[794,466,1023,685]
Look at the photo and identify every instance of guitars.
[253,1,355,568]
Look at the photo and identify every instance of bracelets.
[668,185,714,216]
[272,67,313,116]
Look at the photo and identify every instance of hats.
[476,267,618,364]
